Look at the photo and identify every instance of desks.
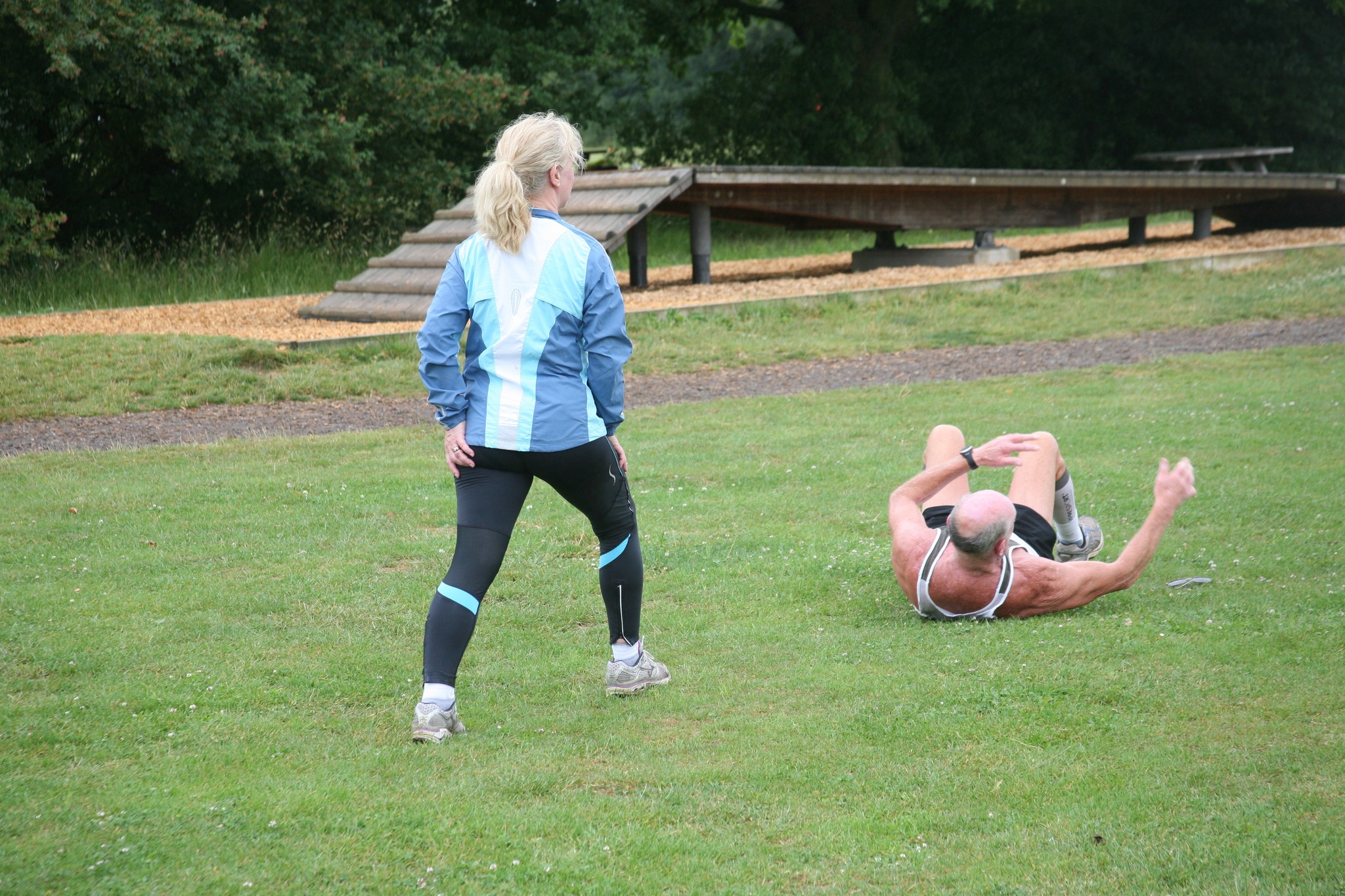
[1127,144,1296,175]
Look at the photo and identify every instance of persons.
[889,423,1199,620]
[412,112,673,742]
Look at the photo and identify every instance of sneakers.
[410,700,467,744]
[605,635,671,698]
[1056,516,1105,564]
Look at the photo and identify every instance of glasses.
[1165,576,1212,590]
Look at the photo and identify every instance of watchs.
[959,446,979,470]
